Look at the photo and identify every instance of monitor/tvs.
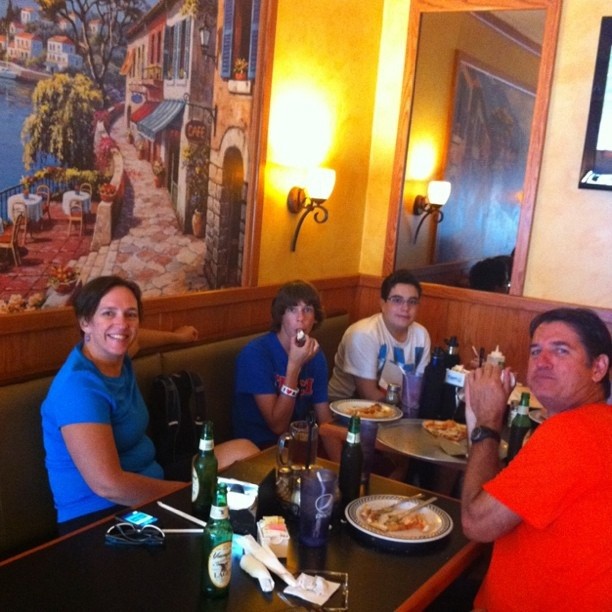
[590,41,611,176]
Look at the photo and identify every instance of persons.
[461,308,612,612]
[328,270,431,417]
[40,276,261,537]
[232,279,343,464]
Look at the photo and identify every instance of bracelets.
[281,384,299,397]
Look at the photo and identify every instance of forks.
[376,491,426,513]
[388,497,439,523]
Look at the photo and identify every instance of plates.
[329,398,404,422]
[375,418,510,465]
[344,493,455,543]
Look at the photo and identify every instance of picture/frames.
[578,16,612,190]
[0,0,279,315]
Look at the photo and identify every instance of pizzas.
[346,403,396,419]
[363,505,428,532]
[421,419,468,443]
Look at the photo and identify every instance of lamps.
[286,168,337,252]
[414,181,451,245]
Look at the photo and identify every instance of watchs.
[471,425,500,444]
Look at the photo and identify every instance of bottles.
[421,347,445,421]
[201,482,235,598]
[337,414,363,524]
[507,392,532,461]
[191,421,219,513]
[437,335,461,420]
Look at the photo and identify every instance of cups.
[277,421,319,470]
[401,370,425,415]
[299,469,337,547]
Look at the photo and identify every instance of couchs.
[0,313,350,535]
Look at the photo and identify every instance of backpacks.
[153,370,208,463]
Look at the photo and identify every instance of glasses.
[387,296,420,307]
[105,522,165,545]
[297,570,348,612]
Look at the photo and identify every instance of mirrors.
[382,0,562,296]
